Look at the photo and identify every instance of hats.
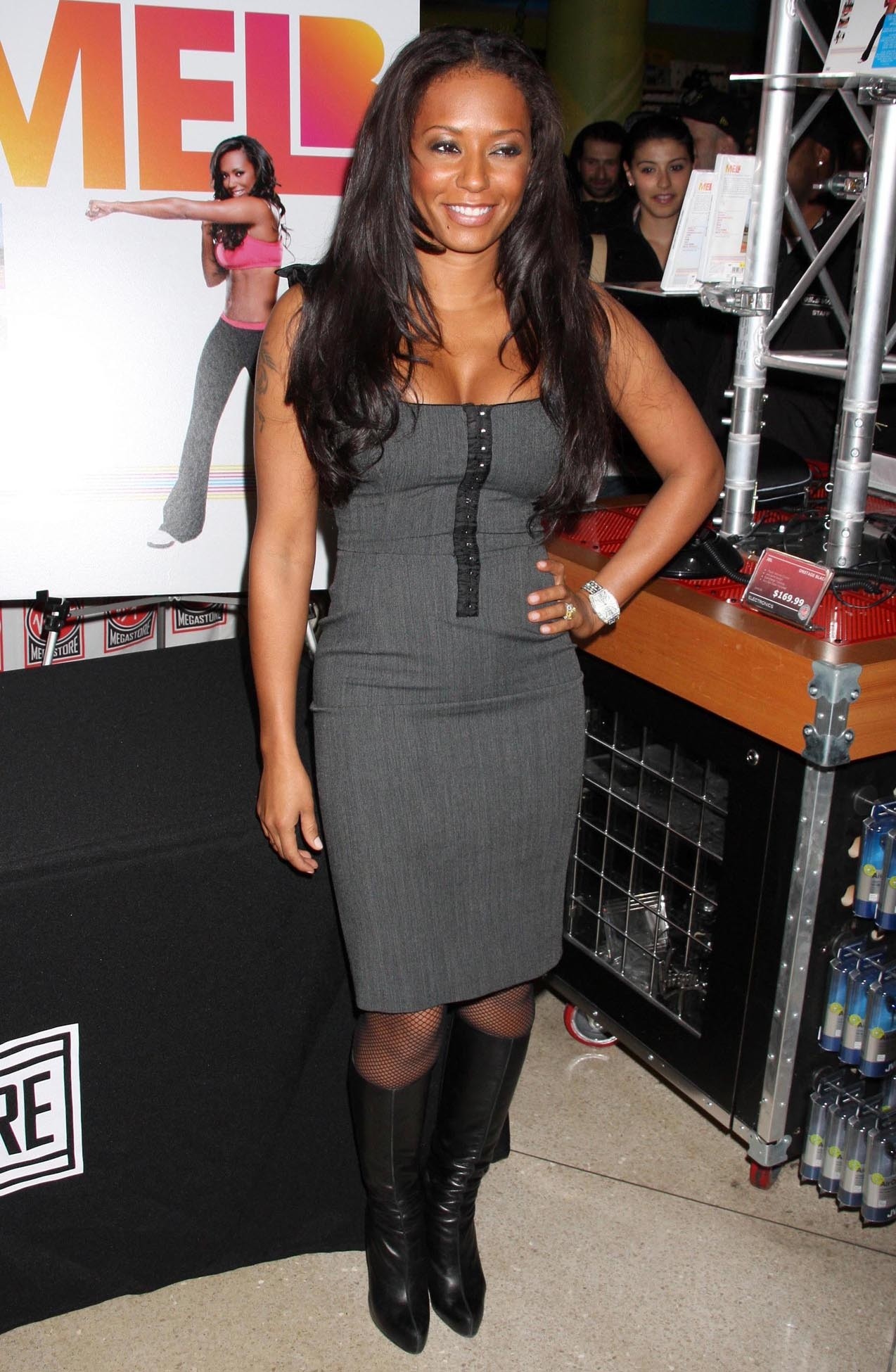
[656,84,745,149]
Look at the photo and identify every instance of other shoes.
[150,527,178,550]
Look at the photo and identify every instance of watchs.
[581,580,620,633]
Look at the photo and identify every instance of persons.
[561,85,896,496]
[249,27,728,1354]
[86,135,289,550]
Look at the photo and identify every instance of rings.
[558,600,576,621]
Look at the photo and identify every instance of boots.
[343,1049,430,1354]
[424,1009,538,1338]
